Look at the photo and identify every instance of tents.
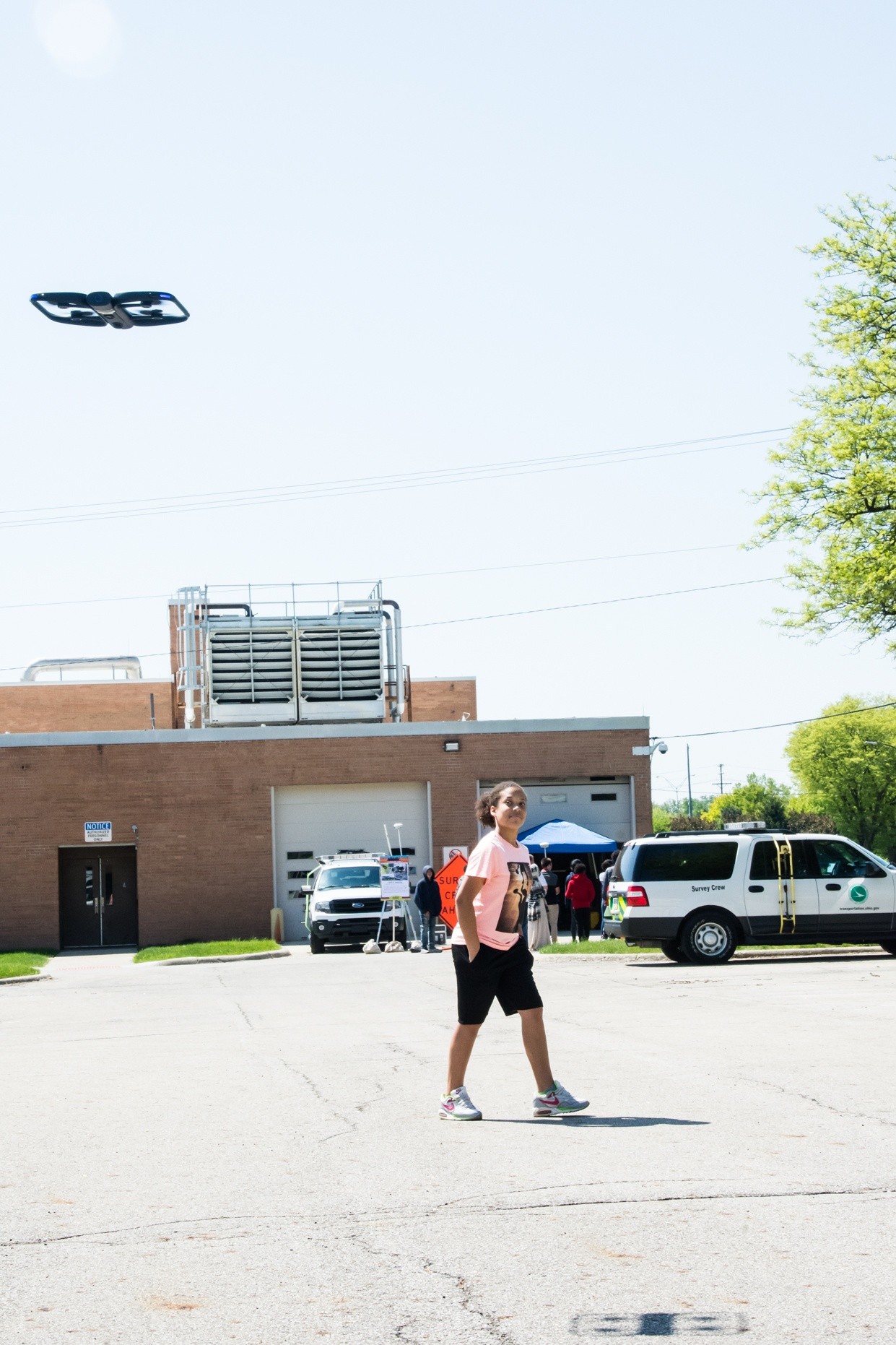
[517,819,618,880]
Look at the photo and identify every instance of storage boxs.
[420,925,446,945]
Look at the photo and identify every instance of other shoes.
[601,935,614,939]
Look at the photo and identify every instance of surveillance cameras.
[659,743,668,754]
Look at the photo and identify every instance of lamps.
[443,740,460,753]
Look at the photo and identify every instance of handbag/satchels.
[384,941,404,952]
[363,939,381,954]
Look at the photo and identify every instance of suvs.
[601,821,896,965]
[303,852,416,955]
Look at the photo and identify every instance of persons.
[540,858,559,944]
[439,781,590,1121]
[414,865,442,954]
[599,850,620,940]
[527,854,551,951]
[565,859,595,943]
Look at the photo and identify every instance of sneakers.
[532,1080,589,1117]
[421,944,442,954]
[439,1086,483,1121]
